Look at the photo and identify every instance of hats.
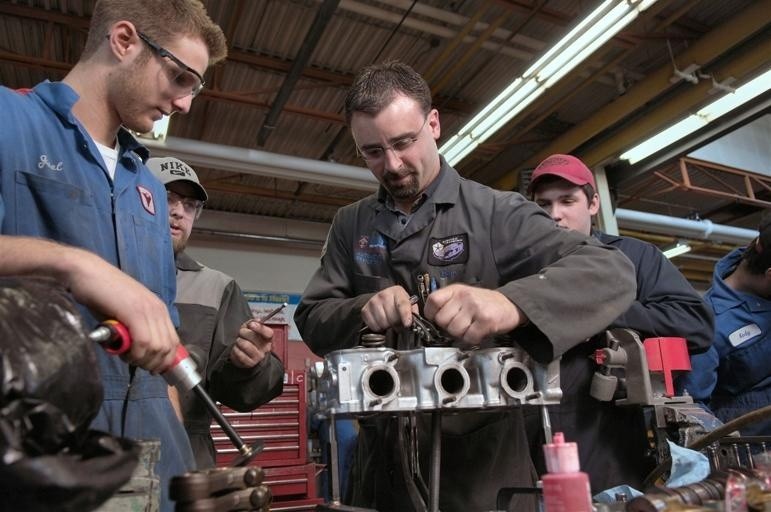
[144,156,209,205]
[526,153,597,194]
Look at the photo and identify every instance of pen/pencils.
[417,270,447,294]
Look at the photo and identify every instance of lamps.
[438,1,655,167]
[619,69,770,166]
[657,234,694,264]
[124,130,397,193]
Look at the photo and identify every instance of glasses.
[105,28,206,102]
[355,110,428,163]
[165,186,205,221]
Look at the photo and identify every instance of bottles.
[535,431,593,512]
[289,369,296,384]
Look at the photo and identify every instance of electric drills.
[88,319,254,458]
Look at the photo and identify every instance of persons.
[0,3,229,512]
[293,56,637,512]
[680,214,770,462]
[503,151,714,492]
[146,155,286,470]
